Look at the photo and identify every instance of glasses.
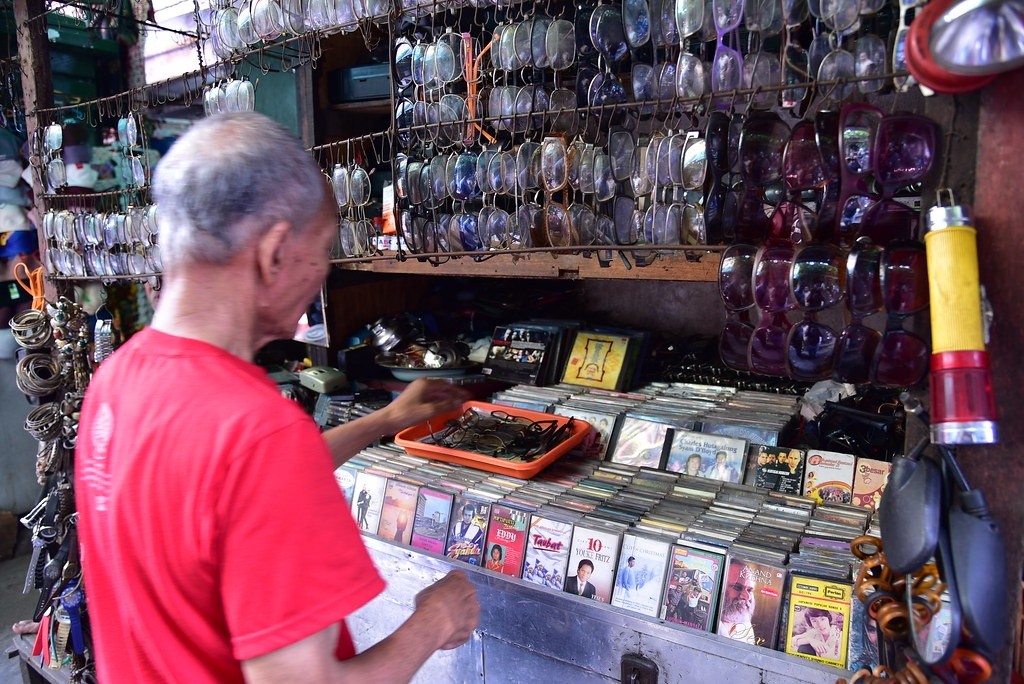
[0,2,963,391]
[425,404,575,464]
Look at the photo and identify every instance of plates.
[373,360,476,381]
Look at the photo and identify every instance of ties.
[580,582,585,594]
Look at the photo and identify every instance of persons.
[681,451,734,483]
[450,504,484,556]
[503,328,537,363]
[752,448,801,493]
[585,416,610,461]
[621,557,636,609]
[806,472,814,496]
[791,607,842,660]
[666,572,703,622]
[357,484,371,530]
[564,559,596,600]
[719,559,755,646]
[487,544,504,573]
[525,562,562,589]
[74,110,478,684]
